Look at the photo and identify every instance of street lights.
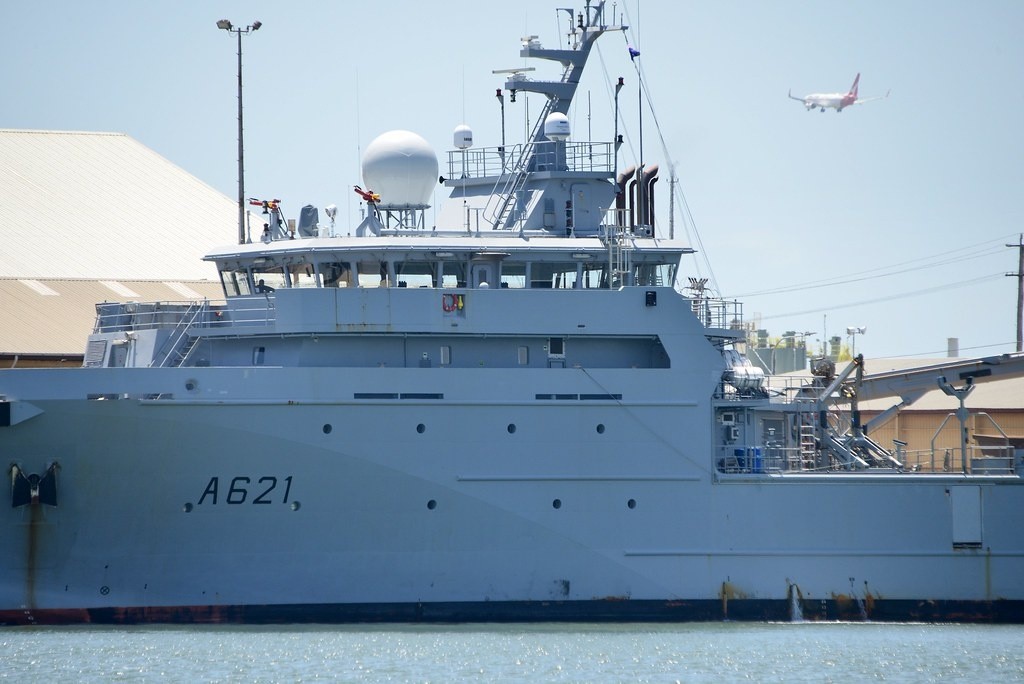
[218,19,263,247]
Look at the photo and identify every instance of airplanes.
[788,73,861,113]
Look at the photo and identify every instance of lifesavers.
[443,293,458,312]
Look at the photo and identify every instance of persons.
[254,278,275,293]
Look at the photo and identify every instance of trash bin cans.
[733,447,762,472]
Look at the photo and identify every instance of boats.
[0,0,1024,625]
